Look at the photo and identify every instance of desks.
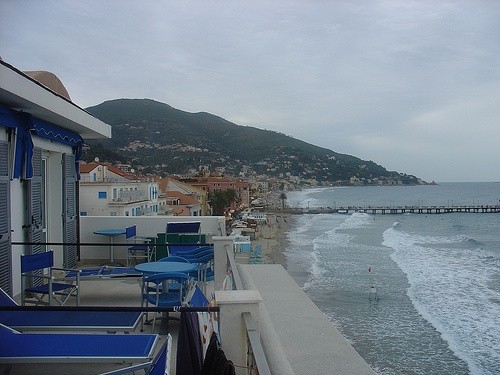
[93,229,126,267]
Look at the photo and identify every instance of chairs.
[20,250,82,307]
[99,333,173,375]
[125,221,215,334]
[62,265,145,278]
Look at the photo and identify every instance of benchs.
[0,288,145,334]
[0,324,160,365]
